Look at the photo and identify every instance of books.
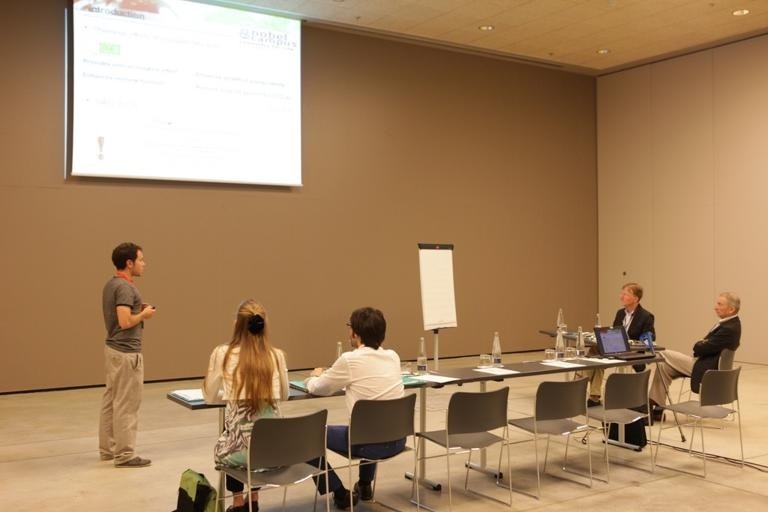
[173,389,204,402]
[290,380,308,394]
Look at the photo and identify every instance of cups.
[545,348,556,360]
[566,347,576,358]
[479,354,490,367]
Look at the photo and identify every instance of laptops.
[593,325,657,360]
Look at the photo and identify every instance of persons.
[304,307,405,509]
[648,291,741,421]
[99,242,156,467]
[200,299,290,512]
[574,282,655,407]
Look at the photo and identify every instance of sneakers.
[226,501,258,512]
[116,456,151,468]
[587,398,601,407]
[653,409,662,416]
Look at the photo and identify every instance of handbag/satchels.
[602,421,646,448]
[177,469,221,512]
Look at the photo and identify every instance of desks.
[167,374,444,512]
[540,331,665,383]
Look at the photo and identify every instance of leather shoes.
[334,489,357,509]
[354,482,372,500]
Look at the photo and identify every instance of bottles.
[576,326,585,358]
[556,327,563,359]
[417,338,428,378]
[593,314,601,329]
[334,340,346,361]
[491,332,501,364]
[556,307,566,333]
